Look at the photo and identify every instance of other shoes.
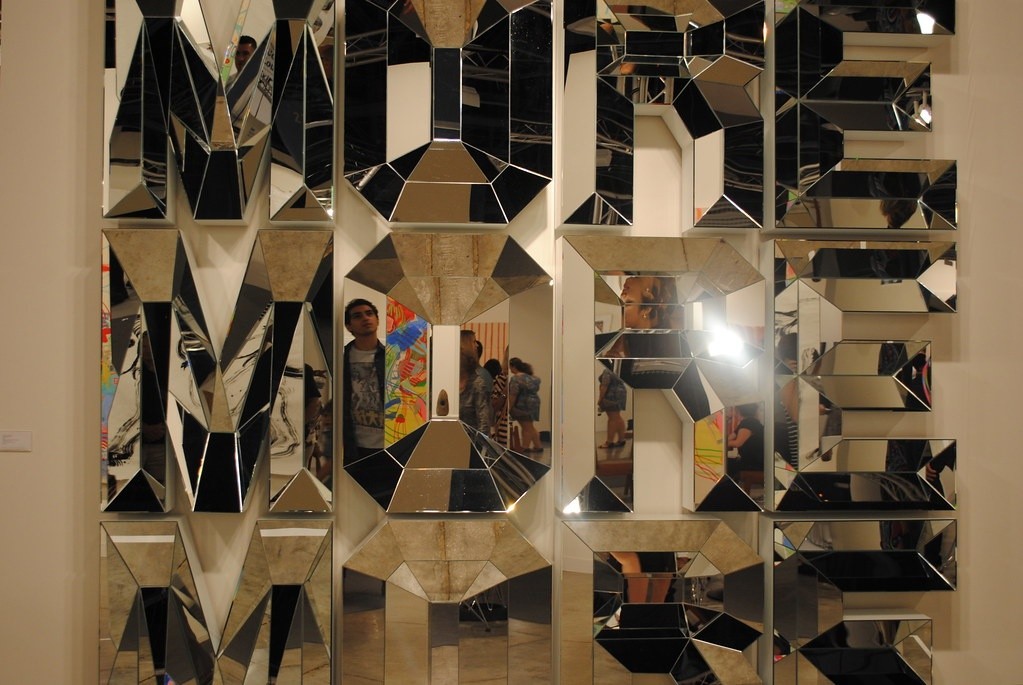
[612,439,626,447]
[523,447,531,452]
[599,442,613,447]
[531,447,544,452]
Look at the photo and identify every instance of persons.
[98,0,975,685]
